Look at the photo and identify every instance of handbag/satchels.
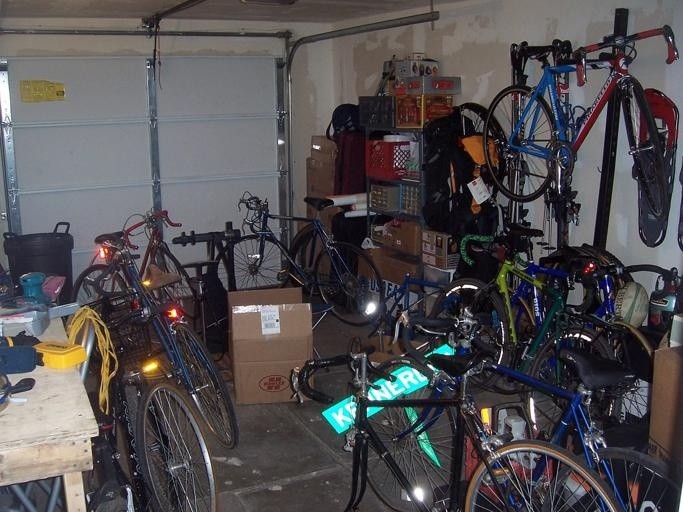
[332,103,359,136]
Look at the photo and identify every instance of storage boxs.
[358,336,425,370]
[647,346,683,480]
[304,55,459,318]
[225,287,316,405]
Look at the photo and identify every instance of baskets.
[86,293,152,369]
[365,140,410,180]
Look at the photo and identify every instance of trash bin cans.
[3,222,73,305]
